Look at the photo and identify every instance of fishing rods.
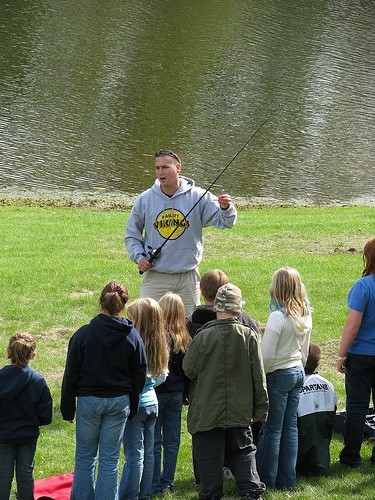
[138,104,281,275]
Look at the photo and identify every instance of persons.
[117,297,171,500]
[150,290,193,496]
[182,284,269,500]
[297,345,336,477]
[124,149,237,323]
[60,281,147,500]
[256,266,312,491]
[337,238,375,467]
[182,268,261,406]
[0,332,52,500]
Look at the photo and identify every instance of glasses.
[155,150,180,164]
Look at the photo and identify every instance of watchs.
[336,355,347,361]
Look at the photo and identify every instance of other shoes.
[240,493,264,500]
[339,459,360,468]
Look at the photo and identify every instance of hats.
[213,283,243,315]
